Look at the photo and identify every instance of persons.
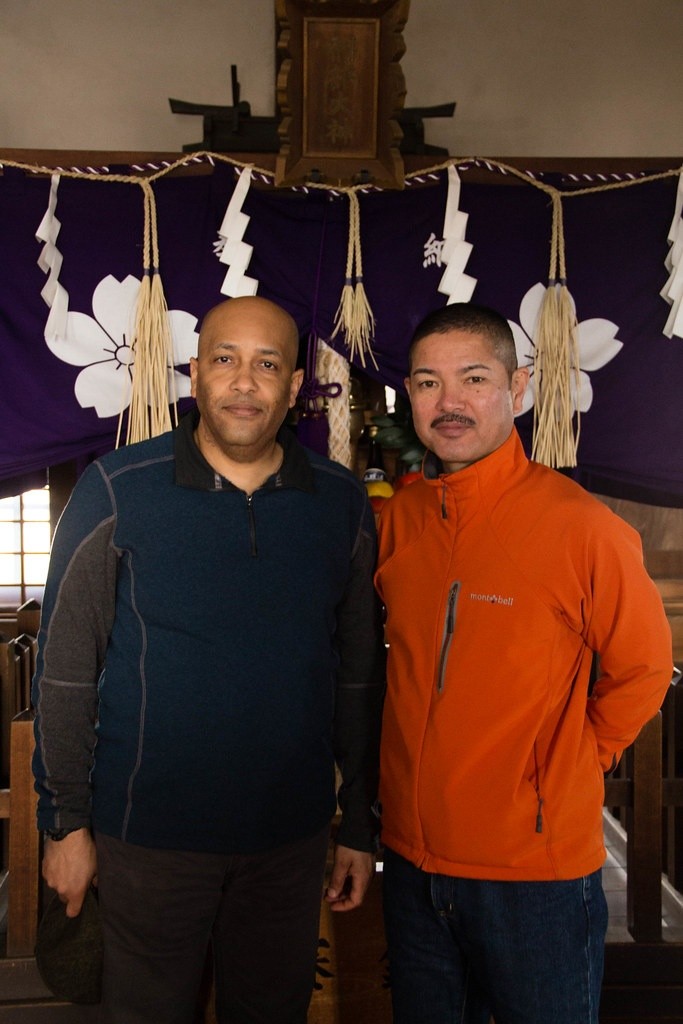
[31,295,378,1024]
[372,301,674,1024]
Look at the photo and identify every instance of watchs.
[46,826,81,841]
